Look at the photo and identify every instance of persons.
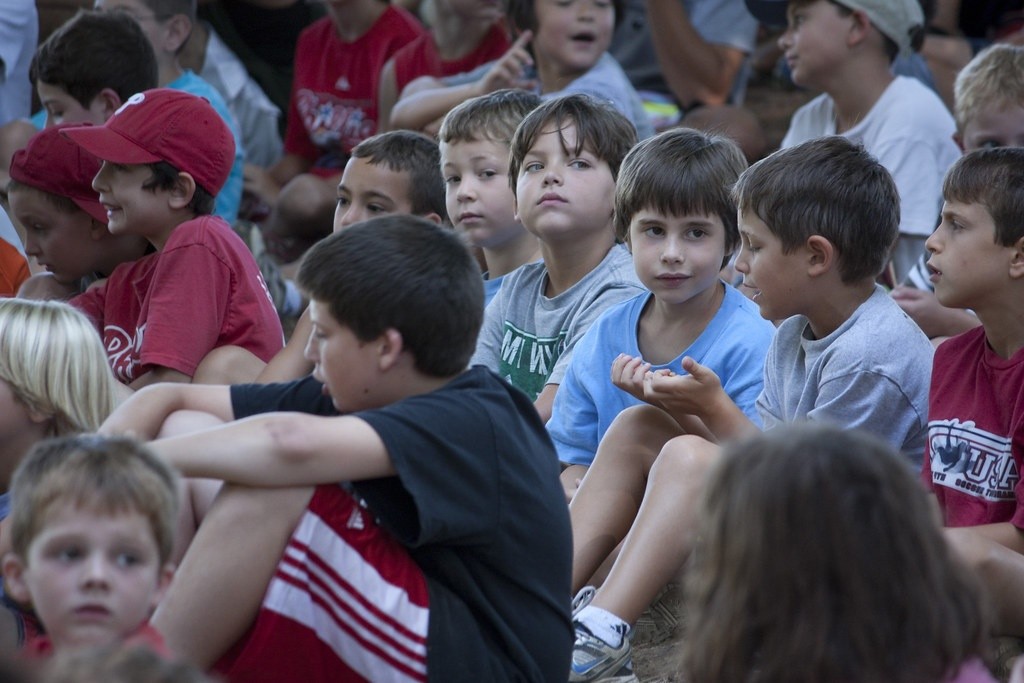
[676,418,1003,683]
[567,137,935,683]
[191,1,1024,641]
[91,215,573,682]
[0,0,282,683]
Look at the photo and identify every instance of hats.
[745,0,925,59]
[9,121,111,224]
[57,88,235,197]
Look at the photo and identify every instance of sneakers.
[564,586,640,683]
